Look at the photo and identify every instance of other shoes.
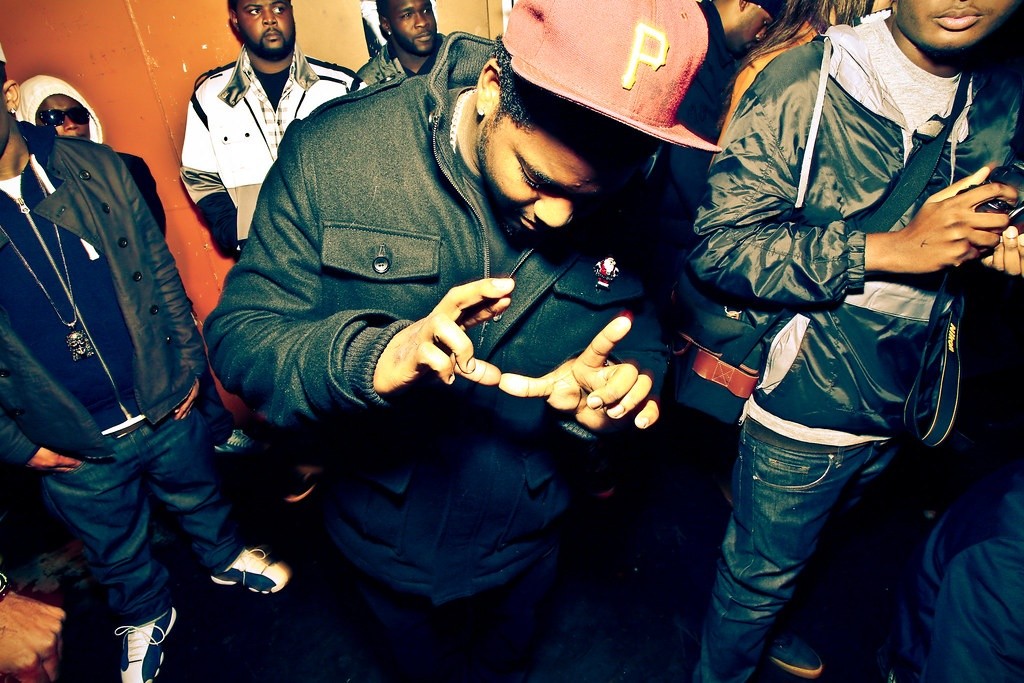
[283,462,323,502]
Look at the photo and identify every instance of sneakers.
[115,605,178,683]
[214,429,255,453]
[761,624,822,679]
[211,546,291,593]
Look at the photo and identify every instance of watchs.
[0,571,16,602]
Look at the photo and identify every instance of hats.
[744,0,785,21]
[500,0,723,154]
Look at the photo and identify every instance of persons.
[352,0,445,85]
[12,73,268,456]
[0,45,291,683]
[202,0,723,542]
[647,0,875,272]
[874,460,1024,683]
[685,0,1024,683]
[180,0,356,502]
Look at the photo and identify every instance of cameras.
[954,164,1024,236]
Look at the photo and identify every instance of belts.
[106,416,147,440]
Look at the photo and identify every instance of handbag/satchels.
[651,261,762,426]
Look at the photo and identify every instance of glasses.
[34,107,90,127]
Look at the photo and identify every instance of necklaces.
[0,154,95,362]
[449,89,479,153]
